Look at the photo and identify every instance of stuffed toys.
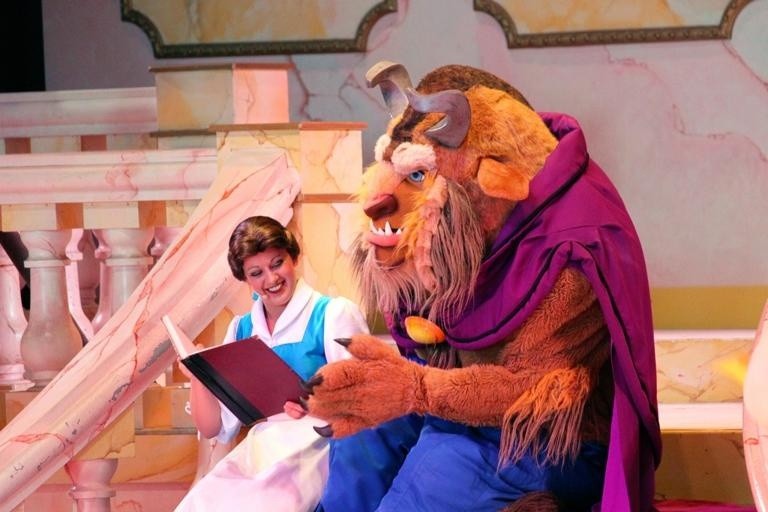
[308,61,661,511]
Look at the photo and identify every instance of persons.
[177,217,370,511]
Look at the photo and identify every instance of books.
[162,315,312,427]
